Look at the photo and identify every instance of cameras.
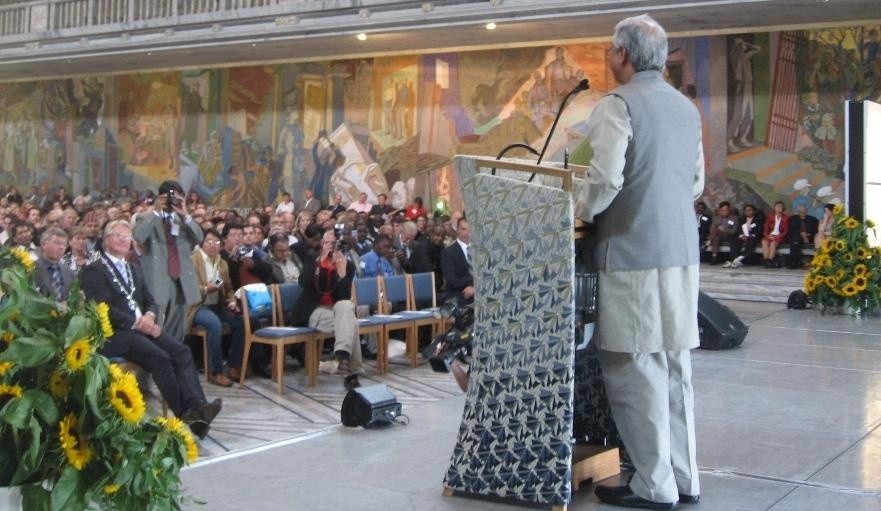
[237,245,252,256]
[331,223,350,253]
[213,278,224,288]
[151,186,177,212]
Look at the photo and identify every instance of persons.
[381,71,417,138]
[568,13,707,511]
[761,201,789,268]
[707,200,741,265]
[695,200,714,258]
[1,182,477,442]
[814,203,840,250]
[785,201,820,270]
[514,44,585,124]
[726,37,763,152]
[724,205,766,270]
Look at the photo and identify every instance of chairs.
[187,268,453,393]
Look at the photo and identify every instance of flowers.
[0,242,208,510]
[806,197,881,319]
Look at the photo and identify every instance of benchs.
[700,232,815,266]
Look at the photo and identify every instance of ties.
[376,260,381,274]
[165,222,182,281]
[467,248,474,275]
[117,262,128,287]
[50,265,61,300]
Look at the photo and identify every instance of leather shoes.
[209,366,241,387]
[189,398,222,442]
[335,358,361,389]
[595,474,699,510]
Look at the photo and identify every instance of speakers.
[787,290,808,309]
[341,383,401,428]
[698,290,749,350]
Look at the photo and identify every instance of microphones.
[528,78,590,183]
